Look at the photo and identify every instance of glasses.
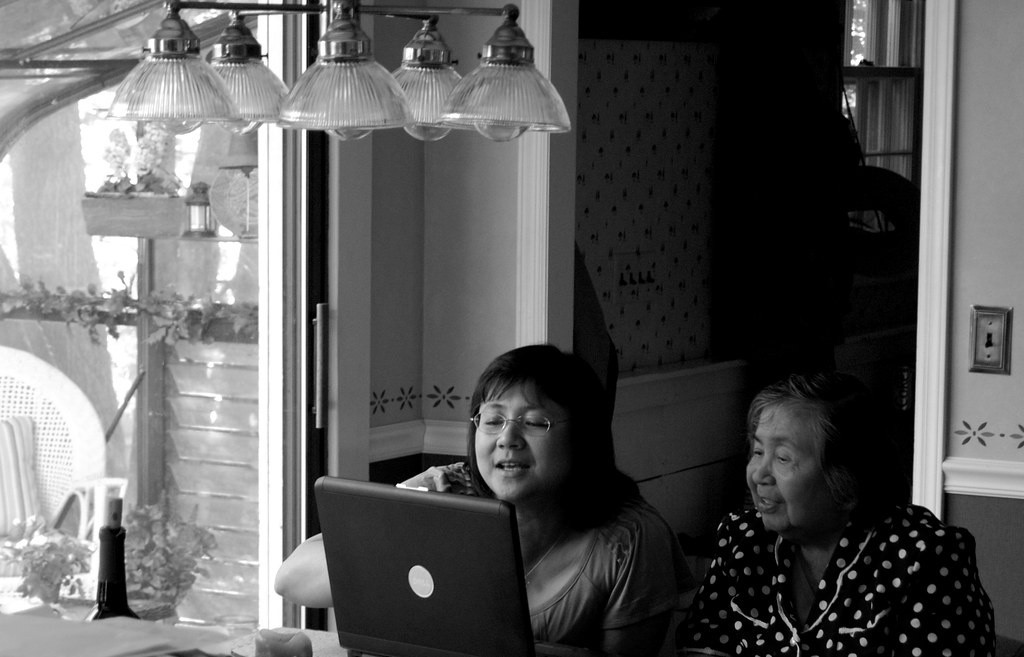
[469,409,569,438]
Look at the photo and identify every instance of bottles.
[82,496,140,622]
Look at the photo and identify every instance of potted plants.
[123,502,215,620]
[5,522,93,607]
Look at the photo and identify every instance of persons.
[677,368,997,657]
[275,344,685,656]
[572,238,620,426]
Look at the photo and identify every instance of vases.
[73,198,186,239]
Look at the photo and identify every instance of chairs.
[0,346,111,577]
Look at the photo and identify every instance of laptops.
[315,476,590,657]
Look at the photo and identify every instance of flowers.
[80,150,187,197]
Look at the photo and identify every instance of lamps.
[107,0,571,143]
